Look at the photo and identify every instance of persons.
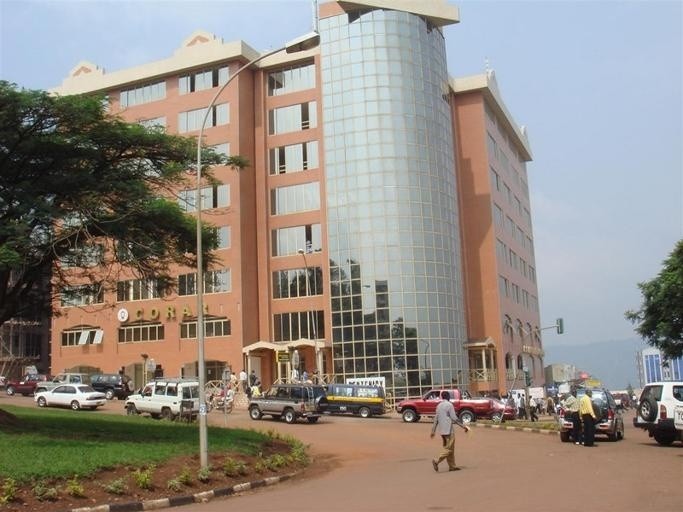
[620,394,639,412]
[563,389,584,444]
[212,369,262,408]
[430,391,468,471]
[291,368,326,385]
[579,389,599,446]
[500,391,559,422]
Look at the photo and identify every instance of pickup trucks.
[398,389,491,424]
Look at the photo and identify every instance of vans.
[321,382,385,419]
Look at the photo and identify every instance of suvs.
[246,381,328,423]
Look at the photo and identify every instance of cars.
[1,370,206,422]
[547,383,683,449]
[464,394,516,424]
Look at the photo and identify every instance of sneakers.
[573,441,598,448]
[431,459,439,472]
[447,466,462,472]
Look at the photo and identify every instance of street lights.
[297,246,321,381]
[190,27,321,472]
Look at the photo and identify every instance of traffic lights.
[590,376,592,382]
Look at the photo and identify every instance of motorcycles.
[205,391,234,414]
